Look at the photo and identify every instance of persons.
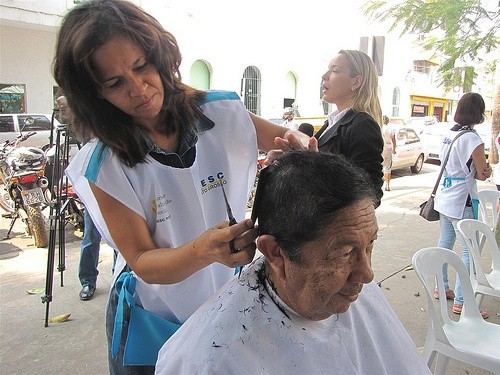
[53,0,325,375]
[154,148,432,375]
[299,122,314,137]
[305,48,386,208]
[76,203,120,301]
[280,108,298,128]
[381,116,397,191]
[428,93,500,319]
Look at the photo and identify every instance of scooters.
[41,136,88,234]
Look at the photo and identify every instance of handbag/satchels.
[419,197,440,222]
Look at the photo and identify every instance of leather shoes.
[80,285,95,300]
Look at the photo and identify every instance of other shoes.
[385,187,390,191]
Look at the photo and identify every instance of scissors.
[221,182,239,253]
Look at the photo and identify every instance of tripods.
[45,126,83,334]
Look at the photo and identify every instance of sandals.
[434,291,455,300]
[452,305,488,319]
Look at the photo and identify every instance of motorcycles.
[0,118,51,249]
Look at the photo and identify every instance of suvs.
[0,114,69,153]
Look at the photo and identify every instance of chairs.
[478,190,500,254]
[412,247,500,375]
[457,219,500,307]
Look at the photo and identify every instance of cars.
[422,122,500,167]
[384,125,429,174]
